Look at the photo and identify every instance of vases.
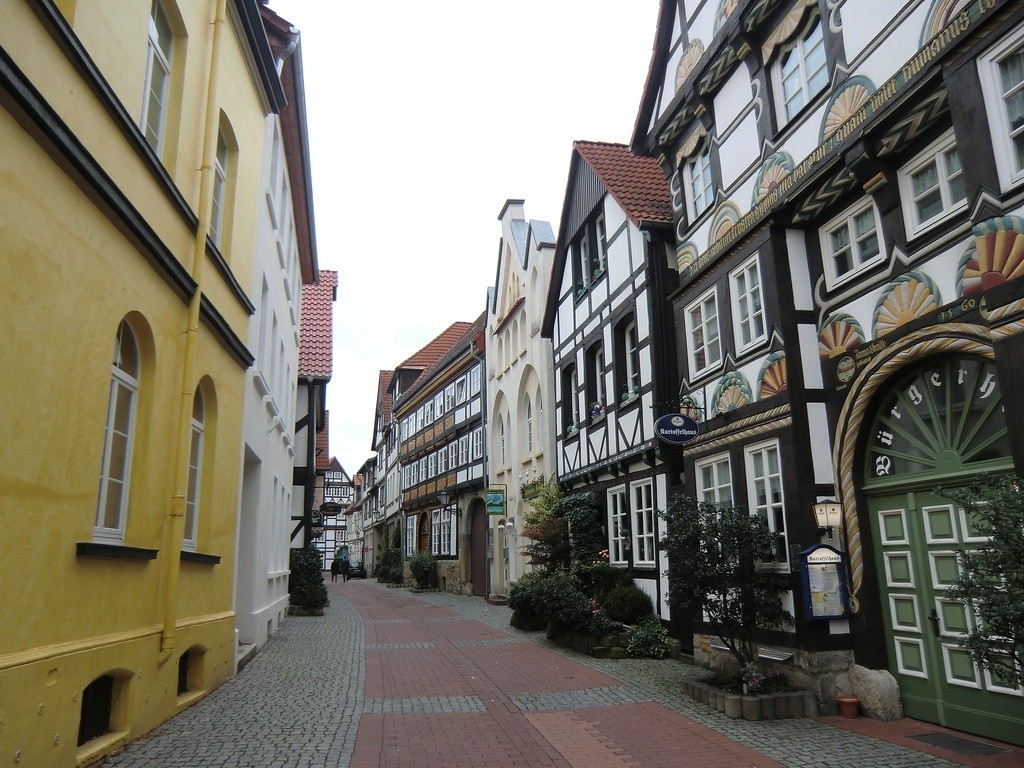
[837,696,860,718]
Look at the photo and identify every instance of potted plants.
[619,384,640,409]
[566,422,579,438]
[587,393,606,426]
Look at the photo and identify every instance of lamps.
[440,486,462,517]
[812,499,846,541]
[497,517,515,531]
[373,509,380,523]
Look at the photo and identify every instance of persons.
[331,559,339,582]
[341,555,349,582]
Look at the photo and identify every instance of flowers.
[746,667,780,692]
